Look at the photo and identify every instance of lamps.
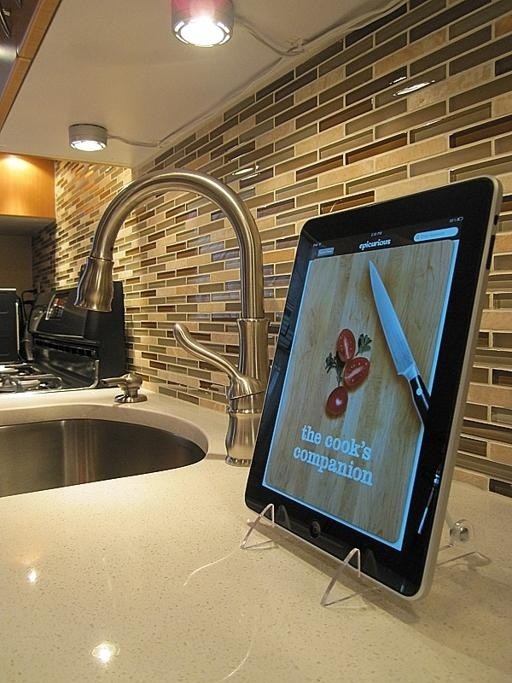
[170,0,303,57]
[68,123,159,152]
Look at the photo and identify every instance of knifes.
[369,259,430,427]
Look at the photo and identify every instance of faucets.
[74,167,272,467]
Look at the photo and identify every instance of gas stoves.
[0,362,91,397]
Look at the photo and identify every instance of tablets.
[244,176,505,603]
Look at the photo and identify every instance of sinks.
[0,402,209,497]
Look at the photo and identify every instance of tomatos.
[326,386,349,415]
[337,328,356,362]
[343,357,369,388]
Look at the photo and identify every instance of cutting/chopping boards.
[266,237,456,545]
